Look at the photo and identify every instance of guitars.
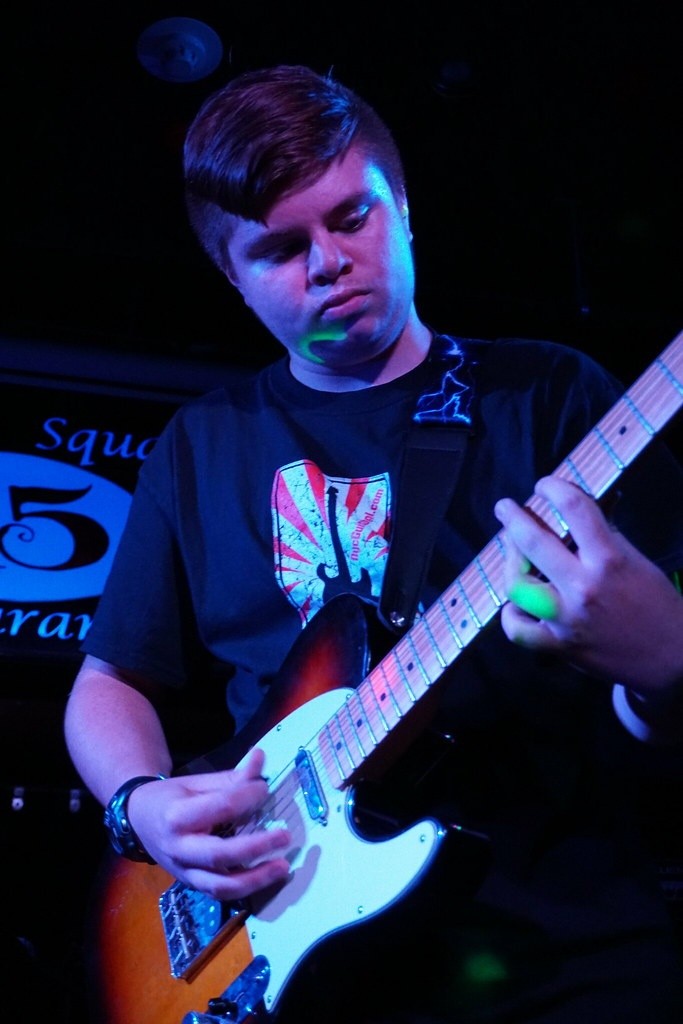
[90,335,683,1023]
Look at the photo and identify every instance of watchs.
[104,772,168,865]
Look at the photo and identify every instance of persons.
[64,66,683,1024]
